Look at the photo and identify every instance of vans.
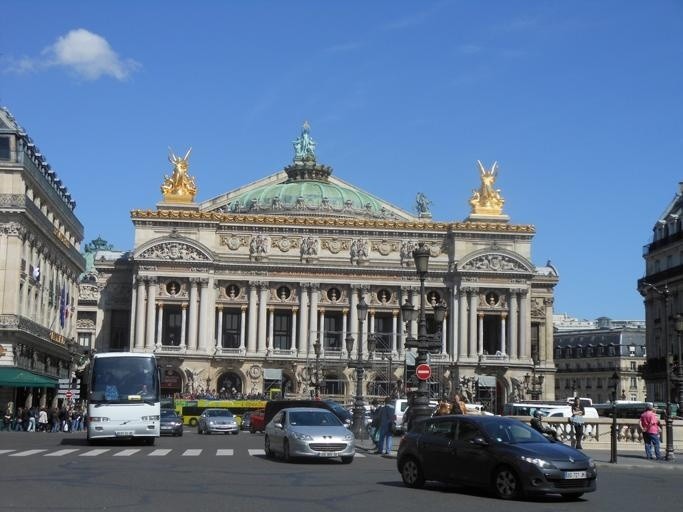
[527,409,600,435]
[263,400,355,432]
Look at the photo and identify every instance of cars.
[249,409,265,433]
[198,408,239,435]
[242,411,253,430]
[265,407,355,464]
[397,413,597,500]
[160,408,184,436]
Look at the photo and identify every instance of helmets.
[534,410,543,417]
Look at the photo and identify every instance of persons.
[0,404,87,433]
[180,387,281,400]
[370,396,396,455]
[572,396,585,449]
[640,403,663,461]
[438,394,468,414]
[137,385,148,396]
[529,410,557,439]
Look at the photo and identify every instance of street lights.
[604,373,627,462]
[639,279,678,461]
[524,350,544,400]
[309,340,326,399]
[672,313,683,416]
[401,242,448,429]
[346,296,379,440]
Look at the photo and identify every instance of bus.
[504,403,599,424]
[173,388,283,430]
[82,351,164,446]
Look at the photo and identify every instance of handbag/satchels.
[389,421,397,433]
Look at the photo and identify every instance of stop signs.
[66,392,73,398]
[416,364,432,380]
